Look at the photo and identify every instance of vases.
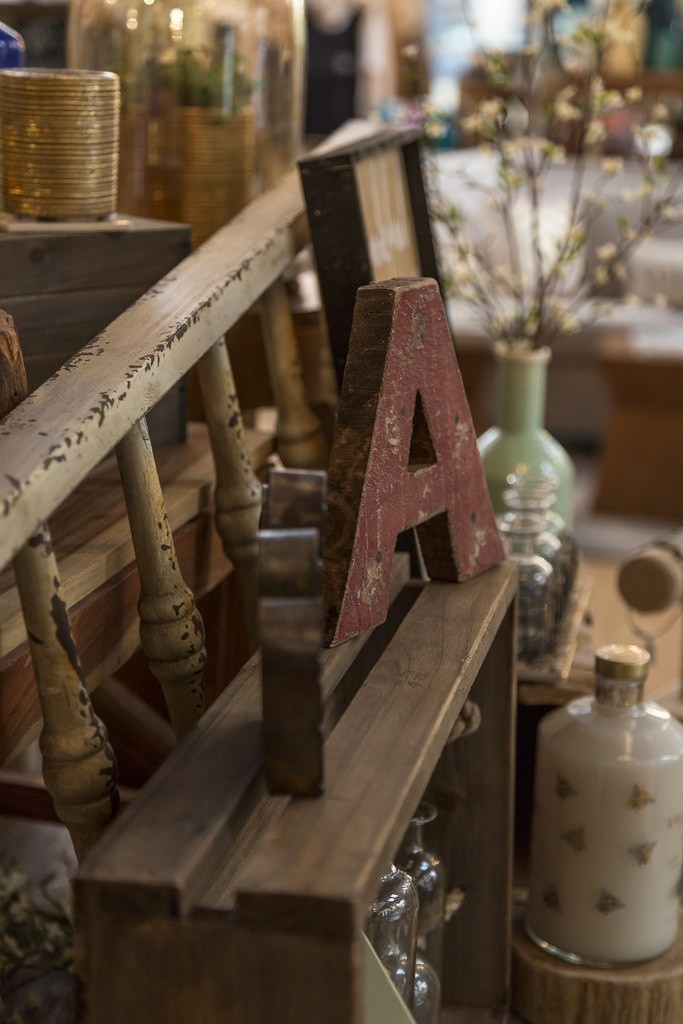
[474,338,577,660]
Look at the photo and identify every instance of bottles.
[361,804,461,1024]
[519,644,682,967]
[496,465,578,656]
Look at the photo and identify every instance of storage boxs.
[0,211,190,462]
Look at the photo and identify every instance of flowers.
[419,0,683,351]
